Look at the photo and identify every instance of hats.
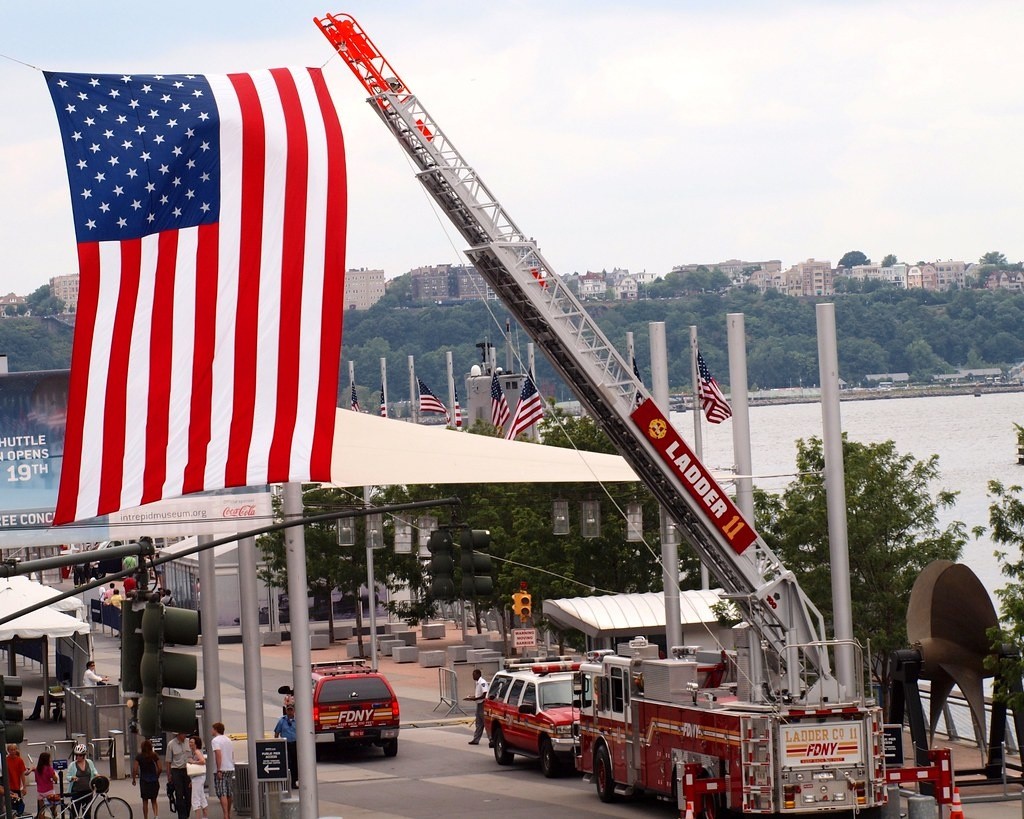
[286,704,294,710]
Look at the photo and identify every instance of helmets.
[74,744,87,754]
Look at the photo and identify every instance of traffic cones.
[949,787,965,819]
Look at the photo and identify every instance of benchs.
[262,631,281,644]
[310,622,555,667]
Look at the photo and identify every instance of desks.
[50,691,66,722]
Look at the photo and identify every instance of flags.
[490,370,511,436]
[42,65,348,527]
[350,376,464,427]
[505,365,544,440]
[696,347,734,425]
[631,357,644,407]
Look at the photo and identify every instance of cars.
[76,539,139,581]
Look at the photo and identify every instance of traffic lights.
[137,601,200,741]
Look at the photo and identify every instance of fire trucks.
[312,8,891,818]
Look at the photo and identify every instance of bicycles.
[37,784,134,819]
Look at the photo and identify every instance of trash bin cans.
[234,761,291,816]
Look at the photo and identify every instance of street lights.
[459,530,492,597]
[518,594,531,618]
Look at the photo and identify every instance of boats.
[974,389,981,397]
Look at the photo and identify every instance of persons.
[468,669,493,748]
[274,703,301,789]
[59,542,200,646]
[0,661,235,819]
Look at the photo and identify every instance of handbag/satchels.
[186,750,207,777]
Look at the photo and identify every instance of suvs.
[310,659,400,757]
[484,654,582,781]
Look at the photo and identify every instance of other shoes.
[25,714,40,720]
[468,741,479,745]
